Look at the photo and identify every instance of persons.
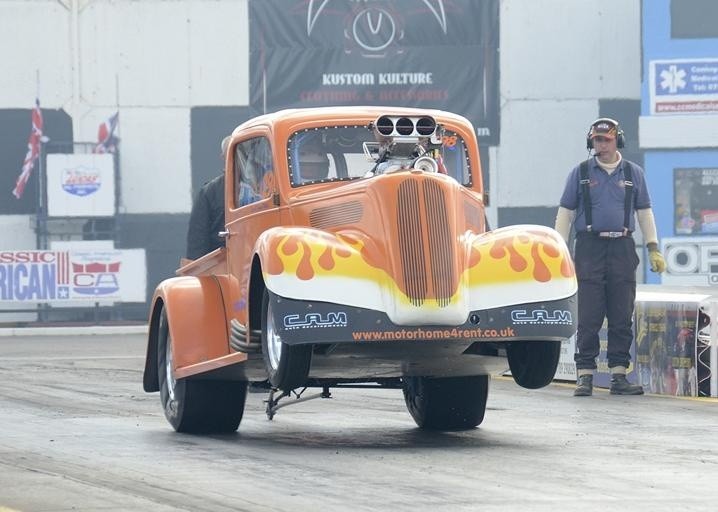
[554,118,667,398]
[290,143,330,184]
[188,135,233,260]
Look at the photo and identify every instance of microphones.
[587,147,605,158]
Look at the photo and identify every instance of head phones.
[587,117,624,148]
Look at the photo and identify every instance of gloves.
[647,248,665,275]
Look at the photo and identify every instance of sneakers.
[609,374,644,396]
[572,373,592,397]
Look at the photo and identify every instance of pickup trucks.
[143,106,580,435]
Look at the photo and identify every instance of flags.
[90,110,120,154]
[11,99,44,199]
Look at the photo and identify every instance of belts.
[590,230,628,238]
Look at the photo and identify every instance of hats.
[587,120,617,140]
